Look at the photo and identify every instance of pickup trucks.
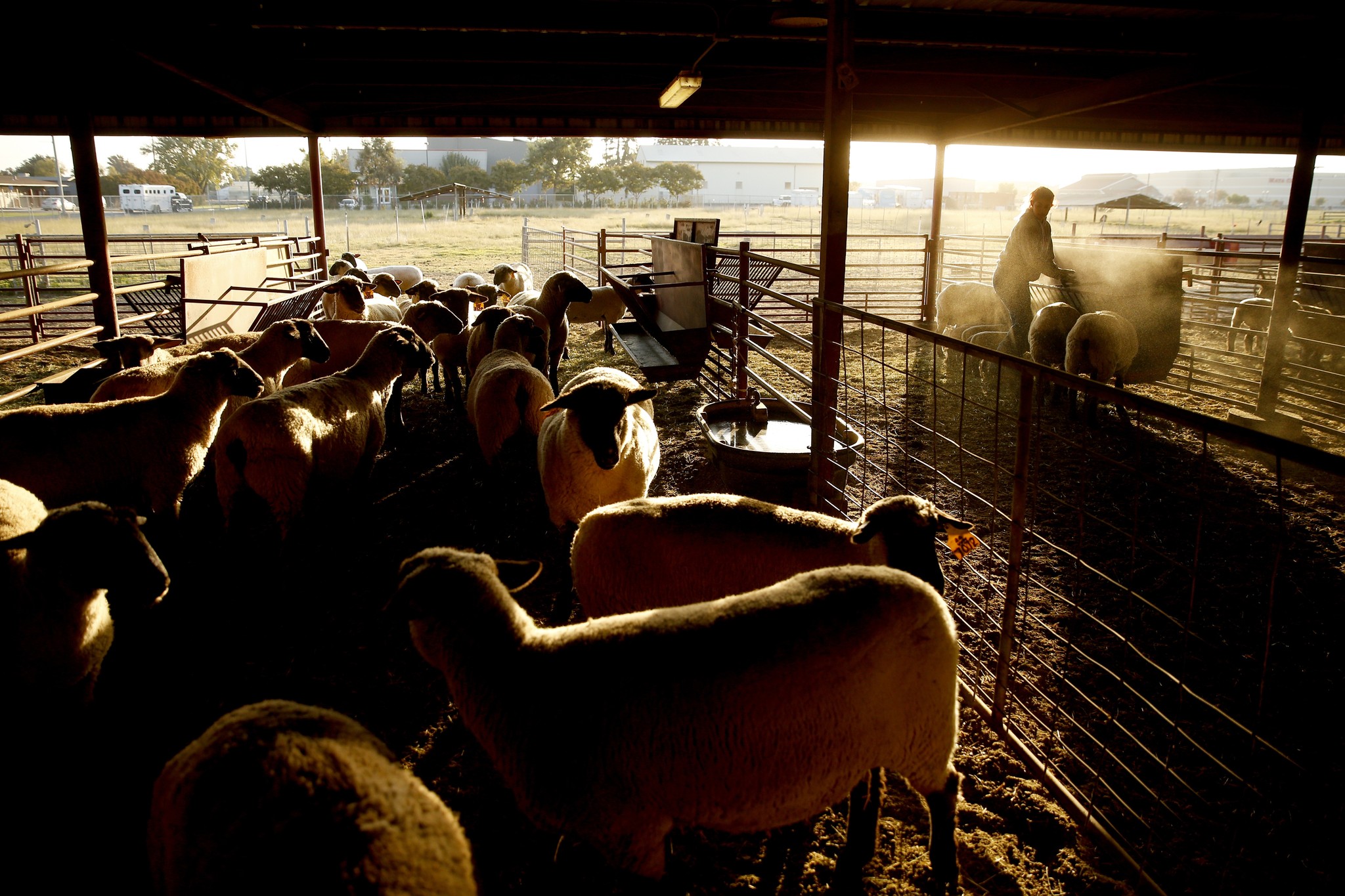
[772,195,791,207]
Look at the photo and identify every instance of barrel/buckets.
[696,397,865,494]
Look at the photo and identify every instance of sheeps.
[1226,295,1332,368]
[148,700,476,895]
[0,252,592,710]
[1027,301,1082,403]
[373,541,961,895]
[537,364,664,530]
[562,273,657,359]
[933,281,1011,368]
[1066,309,1140,421]
[572,494,974,618]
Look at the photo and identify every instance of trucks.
[118,182,181,215]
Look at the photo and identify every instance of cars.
[338,198,359,210]
[255,195,270,202]
[40,198,77,212]
[175,192,193,211]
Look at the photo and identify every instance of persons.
[992,186,1081,357]
